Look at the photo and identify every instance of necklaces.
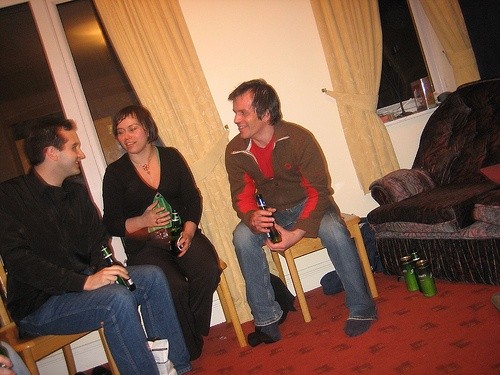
[132,154,154,175]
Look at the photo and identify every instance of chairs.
[265,212,379,323]
[0,252,123,375]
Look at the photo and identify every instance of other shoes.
[344,319,372,338]
[260,323,281,344]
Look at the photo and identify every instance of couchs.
[366,76,500,286]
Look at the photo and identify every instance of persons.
[224,79,377,346]
[1,113,195,375]
[0,340,19,375]
[103,106,220,362]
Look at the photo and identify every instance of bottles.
[100,244,136,292]
[256,193,282,243]
[411,248,420,262]
[169,210,184,254]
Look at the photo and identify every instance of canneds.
[400,256,420,292]
[415,259,437,297]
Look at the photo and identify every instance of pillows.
[479,162,500,185]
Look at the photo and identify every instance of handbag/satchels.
[146,337,177,375]
